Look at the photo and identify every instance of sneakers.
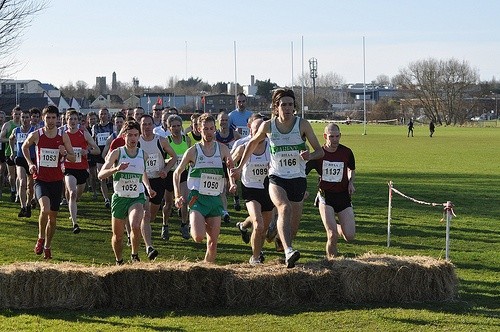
[236,222,250,244]
[43,247,52,260]
[73,224,81,234]
[249,254,263,265]
[145,246,158,260]
[275,235,284,252]
[285,249,301,267]
[266,209,278,244]
[34,237,45,255]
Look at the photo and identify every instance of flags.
[157,97,162,105]
[148,96,151,104]
[202,96,205,104]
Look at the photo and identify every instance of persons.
[0,106,42,218]
[230,89,324,269]
[225,119,274,266]
[40,105,104,235]
[97,115,177,268]
[173,113,238,263]
[209,93,315,243]
[407,119,414,137]
[92,107,167,207]
[21,106,77,259]
[429,121,435,137]
[304,124,355,260]
[153,108,233,240]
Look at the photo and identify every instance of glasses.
[153,108,162,111]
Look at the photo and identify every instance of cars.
[470,112,494,121]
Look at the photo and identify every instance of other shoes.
[115,258,124,266]
[234,197,242,212]
[161,225,170,241]
[130,253,141,263]
[222,212,230,223]
[179,224,191,240]
[104,198,111,207]
[17,205,32,218]
